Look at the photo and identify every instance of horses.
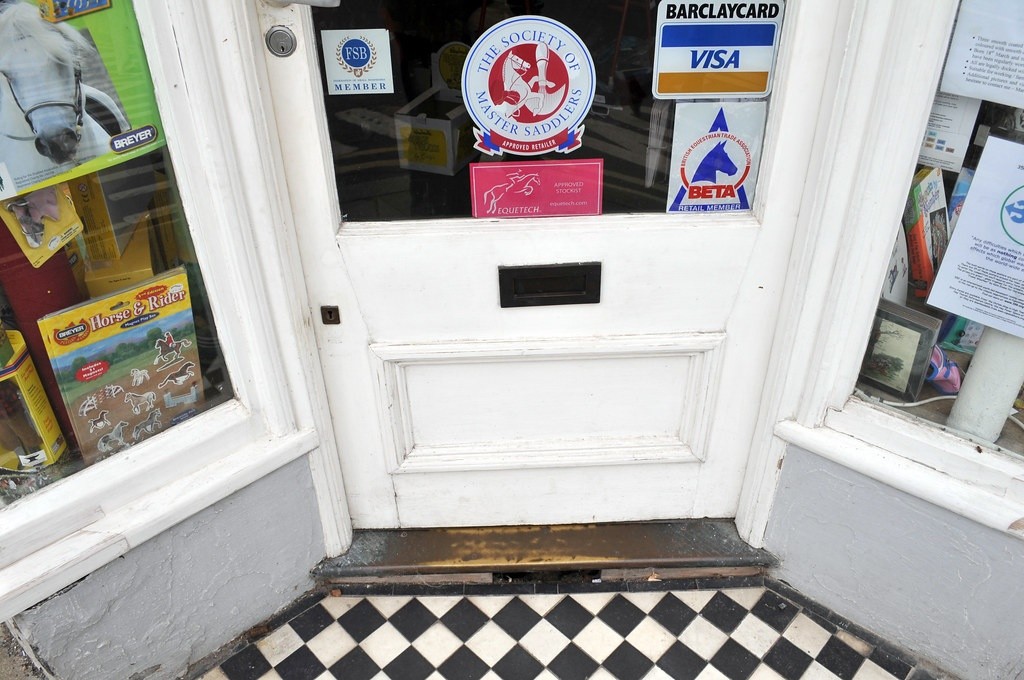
[87,339,195,452]
[0,3,130,171]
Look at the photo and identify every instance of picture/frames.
[857,296,943,403]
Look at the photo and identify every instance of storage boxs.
[903,5,983,298]
[0,152,180,470]
[393,41,482,176]
